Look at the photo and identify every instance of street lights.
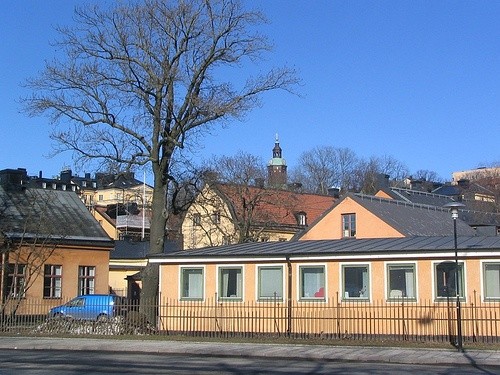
[444,201,469,349]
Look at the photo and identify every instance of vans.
[47,294,128,322]
